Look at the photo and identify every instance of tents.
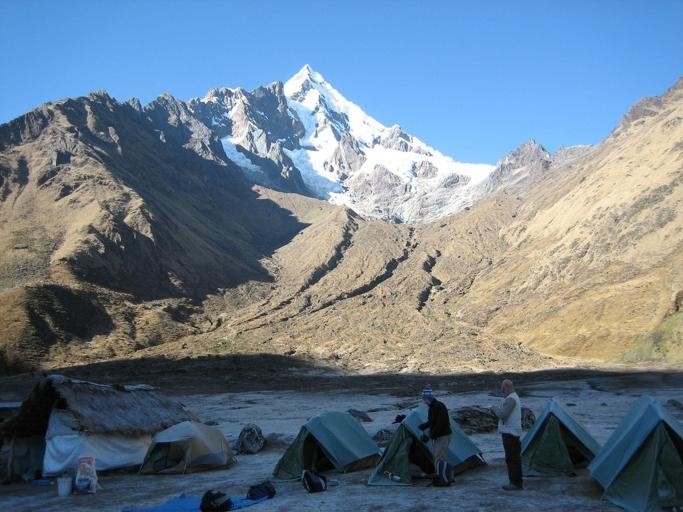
[585,395,683,512]
[267,411,382,482]
[138,421,238,476]
[520,397,604,478]
[367,402,487,487]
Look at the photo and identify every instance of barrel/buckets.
[57,477,73,497]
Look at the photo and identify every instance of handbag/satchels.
[246,481,276,499]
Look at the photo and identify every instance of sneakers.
[502,481,522,490]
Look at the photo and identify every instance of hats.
[421,383,434,399]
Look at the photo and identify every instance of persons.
[488,379,526,490]
[416,385,457,488]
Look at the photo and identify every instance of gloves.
[420,434,429,443]
[418,422,429,430]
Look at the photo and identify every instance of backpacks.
[432,460,454,487]
[301,466,327,492]
[199,489,233,512]
